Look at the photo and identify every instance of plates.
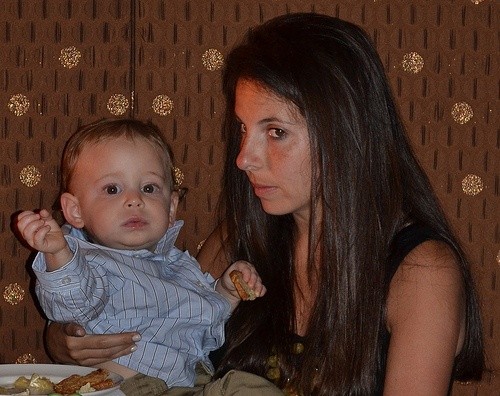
[0,364,125,396]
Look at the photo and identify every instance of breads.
[229,270,256,301]
[54,368,115,394]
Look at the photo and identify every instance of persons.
[17,116,288,396]
[43,12,486,396]
